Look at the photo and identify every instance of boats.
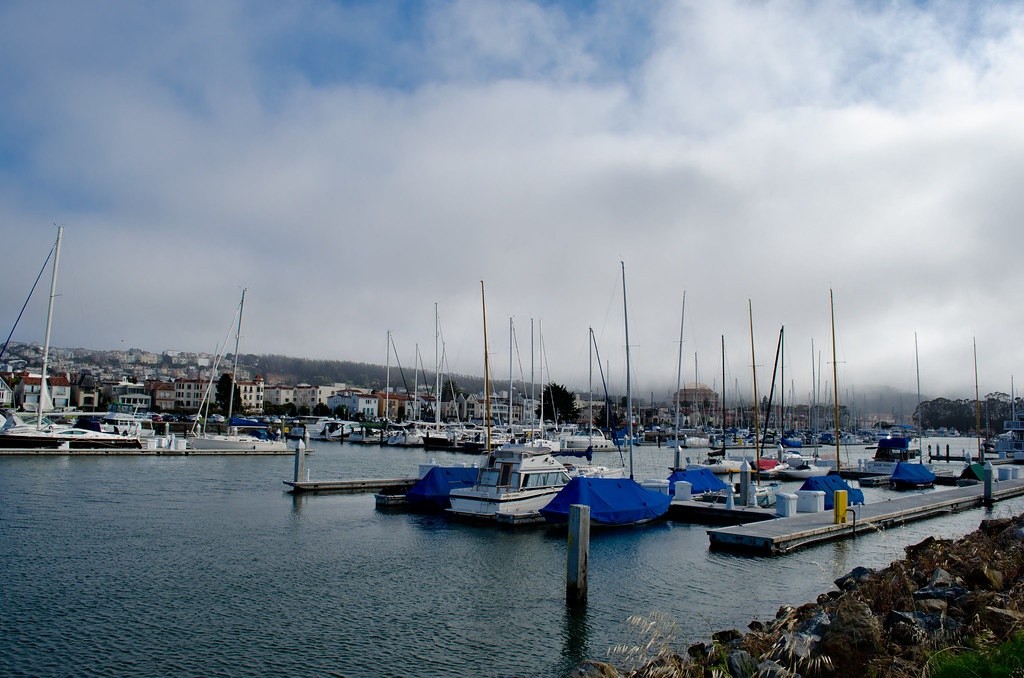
[658,290,1023,525]
[373,464,483,515]
[444,279,627,525]
[537,259,675,529]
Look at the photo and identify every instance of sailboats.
[0,226,1023,462]
